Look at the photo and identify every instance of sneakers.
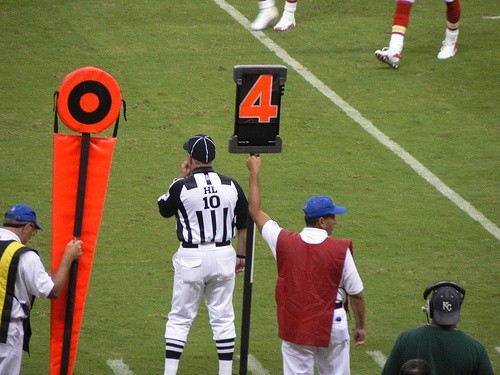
[274,11,295,31]
[437,41,458,59]
[250,6,279,30]
[374,47,401,69]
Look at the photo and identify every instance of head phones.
[422,280,466,321]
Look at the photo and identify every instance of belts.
[182,240,230,248]
[334,301,342,309]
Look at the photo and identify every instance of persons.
[157,134,249,375]
[0,205,83,375]
[374,0,462,70]
[380,280,494,374]
[249,0,299,31]
[247,156,368,375]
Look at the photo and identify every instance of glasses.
[26,223,38,236]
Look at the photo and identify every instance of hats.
[5,204,43,231]
[305,196,345,218]
[432,286,461,325]
[183,135,217,161]
